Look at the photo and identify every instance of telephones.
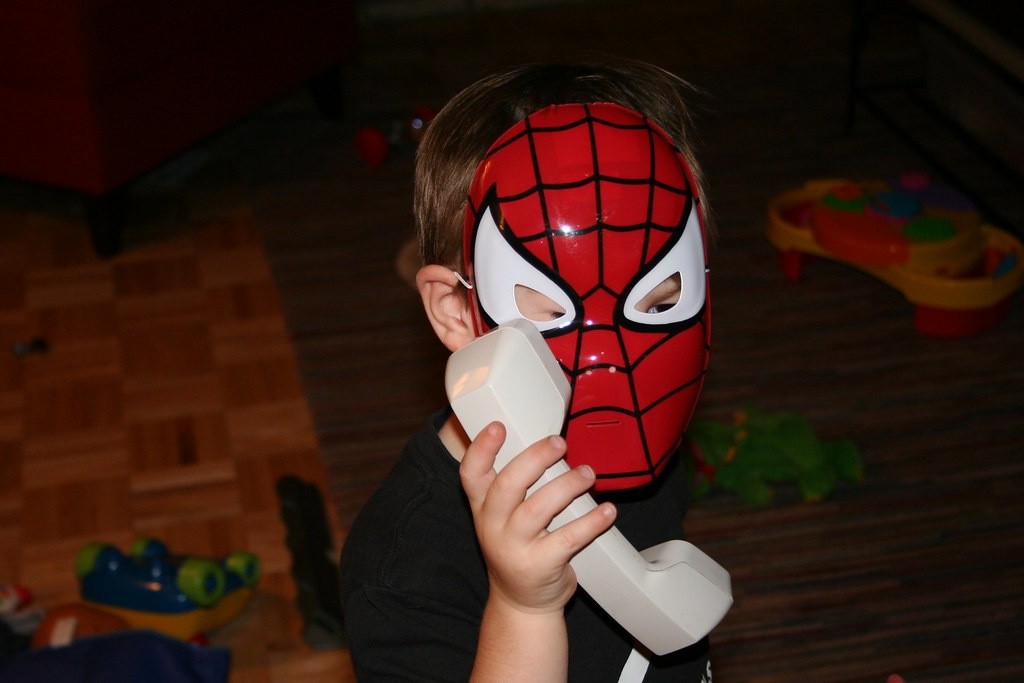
[444,317,734,657]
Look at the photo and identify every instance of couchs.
[0,0,368,259]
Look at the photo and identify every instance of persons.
[336,59,717,683]
